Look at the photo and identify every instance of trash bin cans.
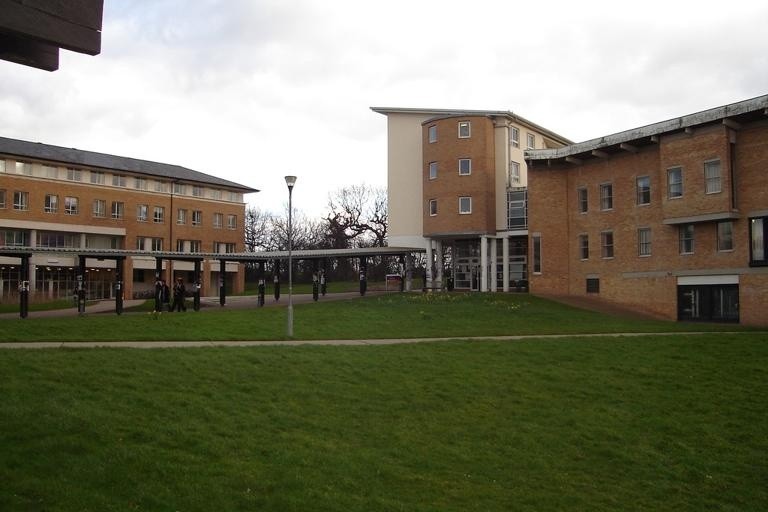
[447,277,454,292]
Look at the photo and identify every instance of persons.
[169,278,187,312]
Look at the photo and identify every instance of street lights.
[284,175,297,334]
